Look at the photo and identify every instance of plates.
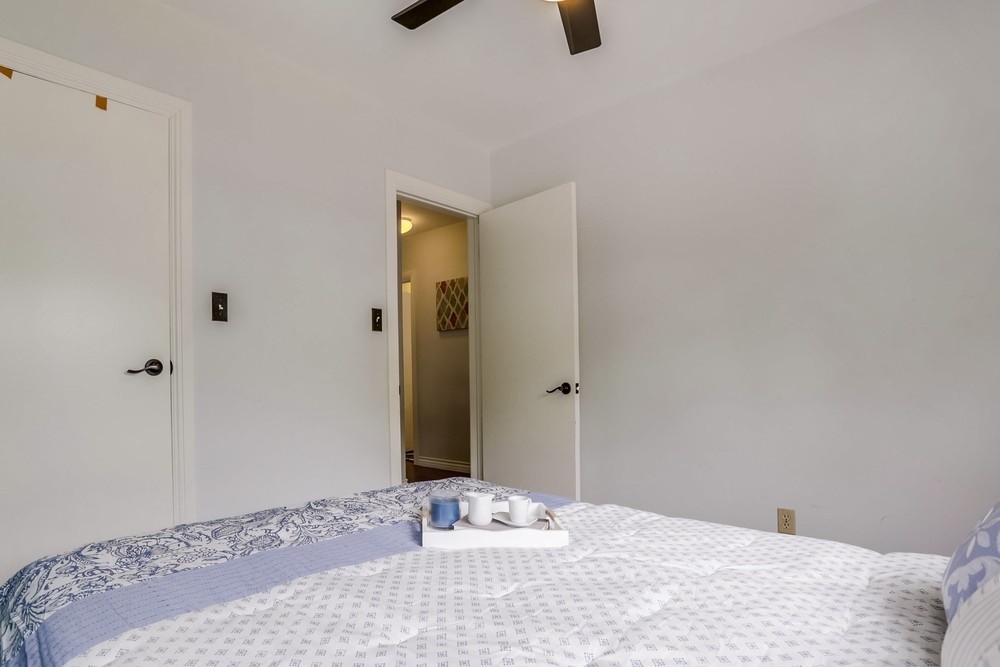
[492,511,538,528]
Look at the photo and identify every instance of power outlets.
[776,507,796,534]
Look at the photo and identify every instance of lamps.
[400,218,412,235]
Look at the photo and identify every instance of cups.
[508,495,532,523]
[468,492,492,526]
[428,489,462,529]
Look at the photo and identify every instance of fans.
[391,0,603,56]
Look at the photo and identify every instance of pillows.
[940,503,1000,667]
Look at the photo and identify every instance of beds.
[0,477,948,667]
